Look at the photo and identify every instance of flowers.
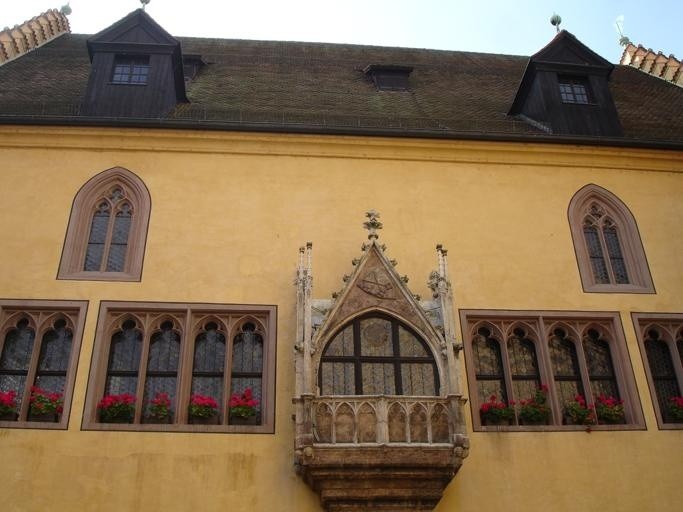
[565,395,595,426]
[518,385,550,420]
[227,387,260,418]
[0,389,19,414]
[96,393,135,413]
[142,393,176,415]
[28,386,63,415]
[479,395,515,419]
[190,395,217,417]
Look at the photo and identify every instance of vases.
[519,416,545,426]
[188,415,220,424]
[139,414,172,424]
[27,411,59,422]
[483,417,509,426]
[101,415,132,424]
[563,414,576,425]
[0,412,18,421]
[228,414,260,425]
